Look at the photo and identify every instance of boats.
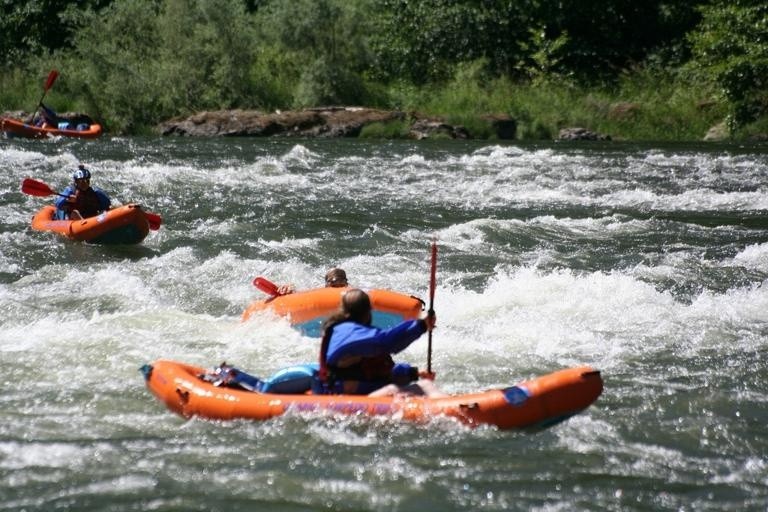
[242,284,426,339]
[5,115,103,143]
[34,202,151,245]
[144,360,603,441]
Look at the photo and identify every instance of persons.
[53,165,111,220]
[30,102,58,129]
[319,289,437,398]
[277,268,348,296]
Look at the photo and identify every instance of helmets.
[73,169,91,180]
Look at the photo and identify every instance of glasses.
[78,178,89,183]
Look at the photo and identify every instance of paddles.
[22,179,162,230]
[28,69,60,128]
[253,278,280,297]
[416,242,436,380]
